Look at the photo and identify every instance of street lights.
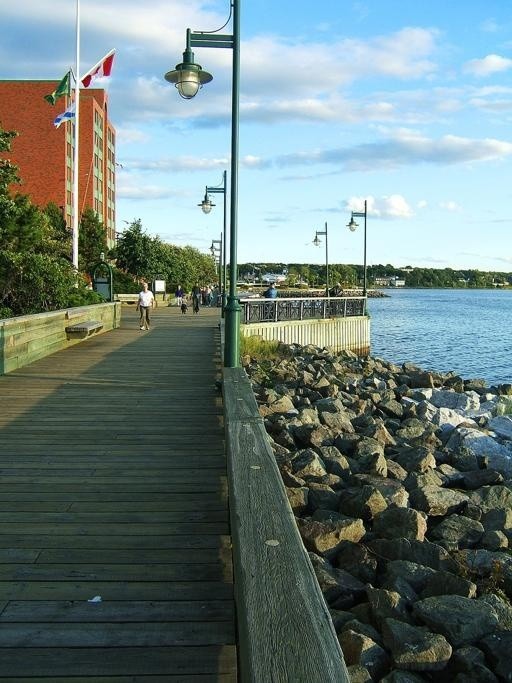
[313,221,330,296]
[164,1,242,367]
[347,198,367,294]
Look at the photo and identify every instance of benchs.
[66,308,105,339]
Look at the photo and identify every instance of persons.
[263,282,277,318]
[175,284,185,306]
[136,282,156,330]
[189,281,202,313]
[328,283,344,295]
[201,283,220,306]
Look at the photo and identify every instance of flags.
[81,51,114,89]
[53,101,77,128]
[45,71,73,105]
